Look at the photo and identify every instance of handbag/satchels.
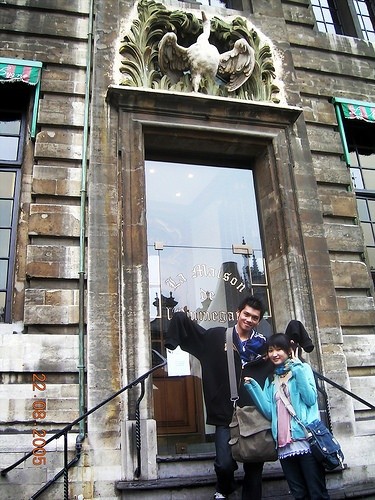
[228,406,279,462]
[304,419,344,470]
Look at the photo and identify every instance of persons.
[244,333,332,500]
[165,296,273,499]
